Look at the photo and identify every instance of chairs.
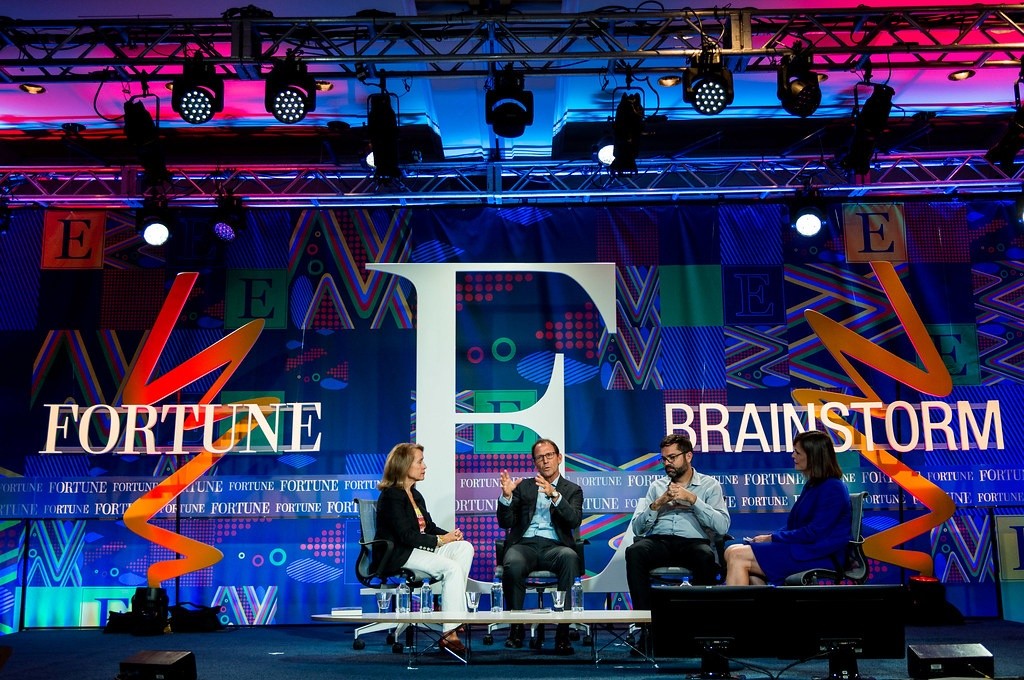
[353,490,868,654]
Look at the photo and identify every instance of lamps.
[171,51,224,124]
[487,61,534,138]
[777,42,1024,175]
[360,70,402,184]
[681,51,733,116]
[134,200,176,247]
[786,184,833,239]
[123,101,170,164]
[211,192,249,242]
[589,64,647,176]
[265,50,316,124]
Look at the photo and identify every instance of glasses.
[658,452,685,464]
[535,452,556,463]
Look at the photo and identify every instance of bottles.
[490,578,503,613]
[570,577,585,613]
[420,578,433,613]
[395,577,411,614]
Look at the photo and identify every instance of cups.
[375,592,392,613]
[466,591,481,612]
[550,591,566,612]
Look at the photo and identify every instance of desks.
[311,609,658,670]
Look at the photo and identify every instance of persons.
[626,434,730,657]
[493,438,586,655]
[369,444,474,655]
[724,430,854,587]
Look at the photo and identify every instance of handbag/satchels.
[103,606,166,635]
[167,601,225,632]
[131,587,169,619]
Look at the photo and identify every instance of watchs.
[550,491,559,498]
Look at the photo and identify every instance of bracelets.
[439,534,445,543]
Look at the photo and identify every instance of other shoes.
[506,625,525,647]
[458,627,464,631]
[555,634,574,652]
[440,639,470,654]
[630,641,645,654]
